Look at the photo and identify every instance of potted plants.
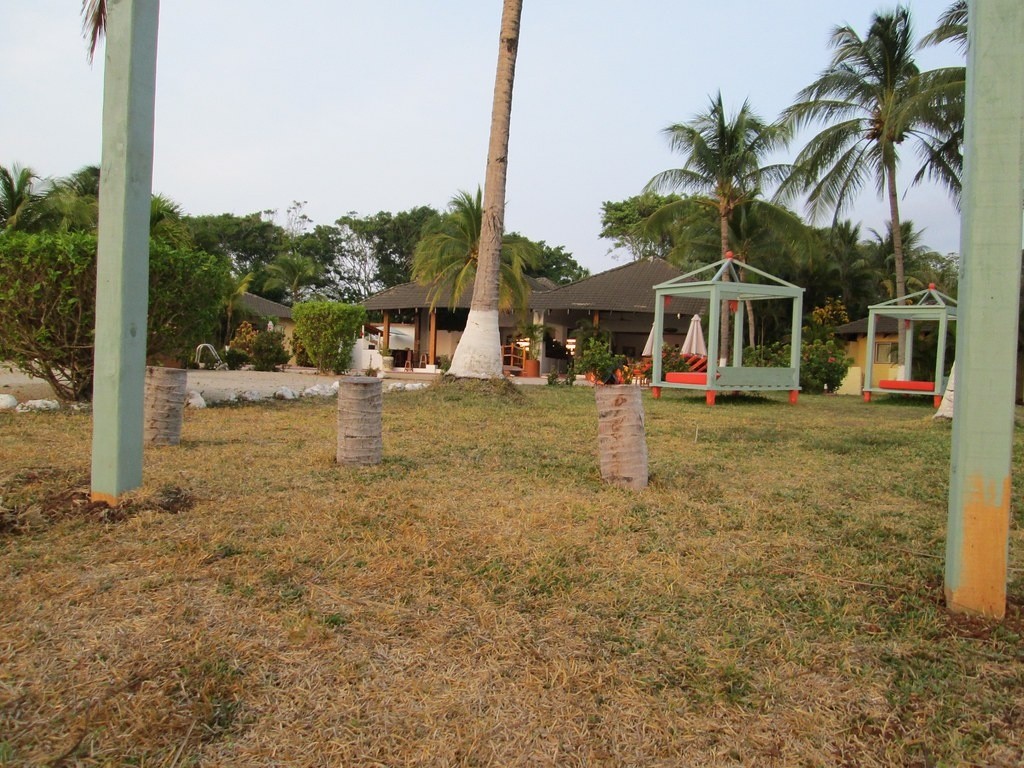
[513,323,554,378]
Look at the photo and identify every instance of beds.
[666,372,721,385]
[878,380,934,391]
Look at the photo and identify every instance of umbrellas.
[641,314,709,358]
